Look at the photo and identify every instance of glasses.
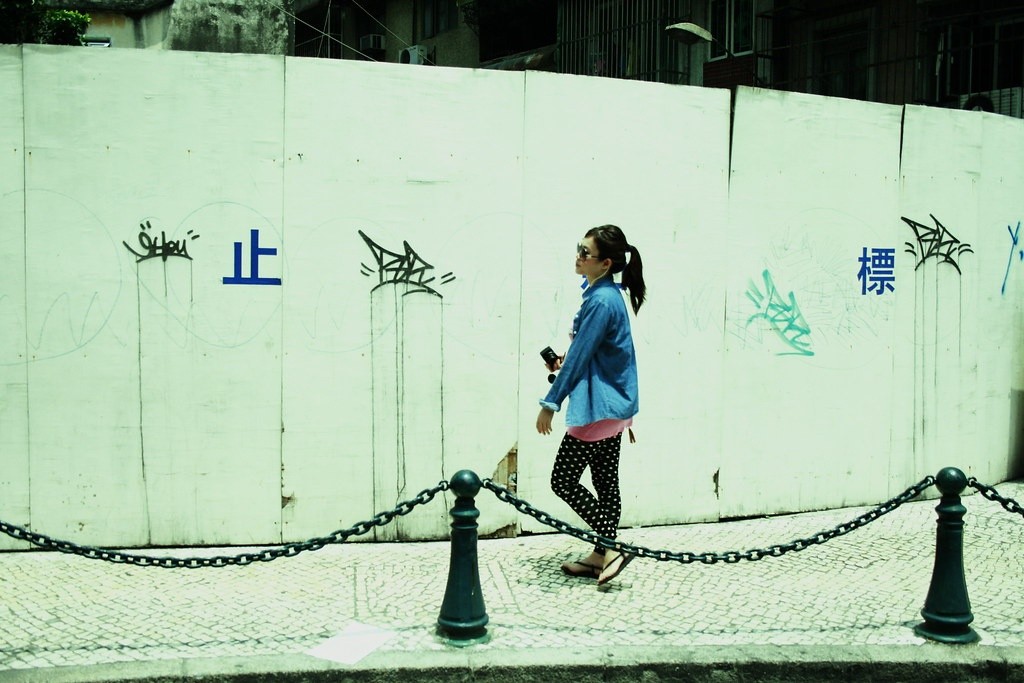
[577,241,598,260]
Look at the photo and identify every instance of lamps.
[360,35,385,48]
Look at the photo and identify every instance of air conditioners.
[960,88,1021,119]
[398,45,427,65]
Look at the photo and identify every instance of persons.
[537,224,646,588]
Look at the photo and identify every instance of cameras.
[540,346,561,369]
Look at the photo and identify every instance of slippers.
[561,561,603,579]
[598,548,638,585]
